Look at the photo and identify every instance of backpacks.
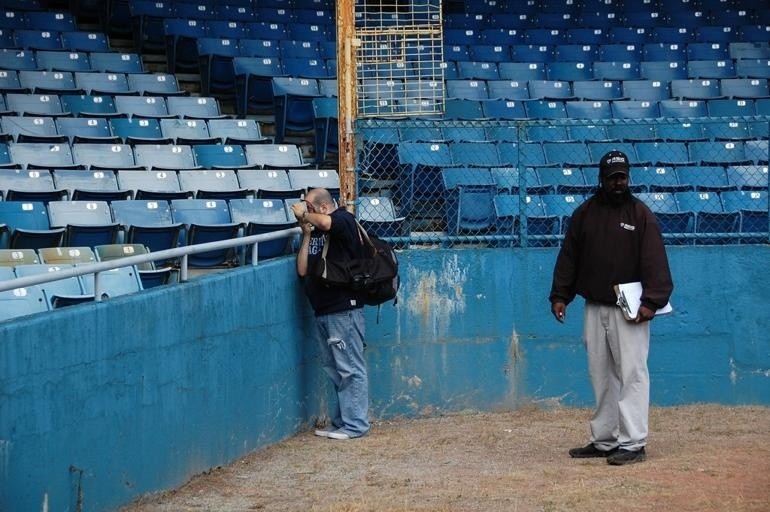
[358,220,401,308]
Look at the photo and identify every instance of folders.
[613,282,672,321]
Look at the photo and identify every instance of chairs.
[38,246,97,264]
[355,0,364,11]
[455,185,496,234]
[284,198,305,221]
[414,61,458,79]
[540,194,587,248]
[398,97,451,121]
[610,100,660,119]
[108,118,176,146]
[662,1,695,16]
[14,264,94,309]
[239,170,306,199]
[193,145,260,169]
[0,30,17,48]
[730,41,770,59]
[567,30,607,45]
[134,145,202,170]
[493,193,560,249]
[443,15,489,29]
[449,141,510,168]
[36,50,91,72]
[0,95,15,115]
[289,23,326,40]
[295,9,332,24]
[511,45,555,63]
[745,122,769,140]
[327,60,374,76]
[525,29,567,45]
[606,124,656,142]
[485,127,518,142]
[313,99,339,160]
[238,40,280,58]
[0,248,40,267]
[737,59,770,79]
[600,45,640,62]
[372,61,415,79]
[320,80,367,98]
[104,0,136,49]
[165,19,207,73]
[488,80,538,101]
[219,5,255,22]
[443,100,497,122]
[1,268,49,323]
[76,262,143,302]
[116,170,193,200]
[720,190,770,245]
[542,0,579,13]
[61,31,111,52]
[408,1,441,15]
[536,168,593,194]
[573,81,630,101]
[232,56,281,113]
[0,48,37,70]
[713,10,755,26]
[468,44,511,62]
[555,45,599,60]
[623,0,658,12]
[133,0,174,55]
[160,119,223,144]
[588,143,639,168]
[580,0,617,12]
[358,196,405,236]
[674,191,742,245]
[19,70,88,96]
[688,141,755,166]
[593,61,641,80]
[755,98,770,115]
[54,171,135,200]
[75,72,140,96]
[363,80,405,97]
[741,25,770,41]
[671,11,711,28]
[671,80,721,101]
[482,101,535,121]
[497,143,546,168]
[635,193,697,245]
[441,46,470,63]
[687,43,728,60]
[687,60,736,79]
[72,0,103,31]
[272,75,325,143]
[178,170,255,200]
[676,165,735,193]
[2,116,69,144]
[361,129,411,145]
[114,94,178,119]
[727,165,769,191]
[695,26,738,44]
[652,123,708,140]
[0,201,65,249]
[0,143,16,168]
[256,8,290,23]
[359,98,408,120]
[441,168,497,195]
[177,0,216,20]
[0,12,29,29]
[288,169,341,198]
[0,168,71,201]
[525,126,569,142]
[166,97,234,120]
[484,28,523,45]
[509,0,541,13]
[447,80,489,100]
[539,13,575,29]
[95,245,172,287]
[55,116,119,143]
[609,26,653,43]
[578,13,619,29]
[629,167,679,193]
[408,80,449,100]
[397,143,462,167]
[246,143,310,168]
[499,61,547,80]
[409,13,443,31]
[566,126,609,144]
[652,27,691,42]
[355,13,370,27]
[207,22,244,40]
[229,199,296,237]
[547,61,593,81]
[641,62,686,79]
[624,11,665,27]
[109,200,178,250]
[583,168,600,194]
[0,70,27,94]
[246,23,284,38]
[18,30,61,51]
[127,73,187,97]
[444,30,483,44]
[745,141,770,165]
[90,52,143,73]
[456,62,500,81]
[720,79,769,99]
[31,13,76,31]
[171,198,249,245]
[707,99,756,117]
[9,143,87,170]
[524,101,579,119]
[281,58,326,76]
[701,123,749,141]
[405,45,442,60]
[197,37,238,95]
[399,126,444,144]
[48,201,129,246]
[464,1,507,14]
[208,120,272,144]
[659,99,707,118]
[565,101,613,119]
[490,14,529,29]
[6,94,71,117]
[73,143,148,170]
[296,0,329,9]
[543,142,593,168]
[439,126,484,143]
[642,43,687,61]
[635,143,699,167]
[491,167,556,196]
[623,80,669,101]
[702,0,735,13]
[62,94,123,118]
[320,42,337,59]
[378,13,408,28]
[528,80,577,100]
[279,39,322,59]
[366,43,391,60]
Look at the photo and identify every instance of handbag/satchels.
[311,218,400,304]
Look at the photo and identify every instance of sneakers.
[569,444,620,457]
[607,445,645,465]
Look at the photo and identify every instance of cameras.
[290,203,316,233]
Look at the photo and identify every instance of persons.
[549,151,674,467]
[292,187,371,438]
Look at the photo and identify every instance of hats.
[599,151,629,177]
[315,425,338,437]
[328,430,349,440]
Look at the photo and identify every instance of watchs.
[301,212,307,221]
